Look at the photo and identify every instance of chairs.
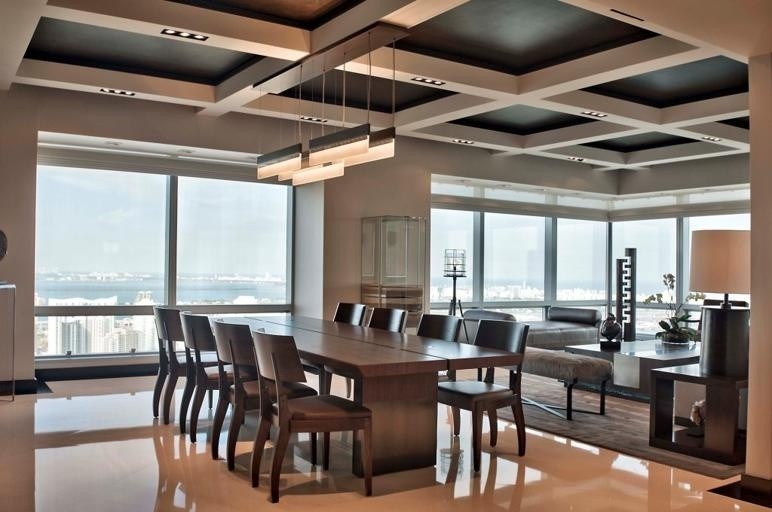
[212,319,317,471]
[438,319,524,471]
[250,328,374,501]
[154,304,526,443]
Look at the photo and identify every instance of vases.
[660,331,690,345]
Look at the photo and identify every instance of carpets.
[439,366,745,480]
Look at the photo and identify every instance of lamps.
[251,23,411,187]
[689,231,752,380]
[444,249,467,316]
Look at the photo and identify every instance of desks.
[648,364,747,466]
[217,319,524,478]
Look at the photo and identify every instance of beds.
[464,307,602,347]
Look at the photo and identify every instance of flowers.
[644,274,707,341]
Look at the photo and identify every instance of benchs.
[494,345,614,421]
